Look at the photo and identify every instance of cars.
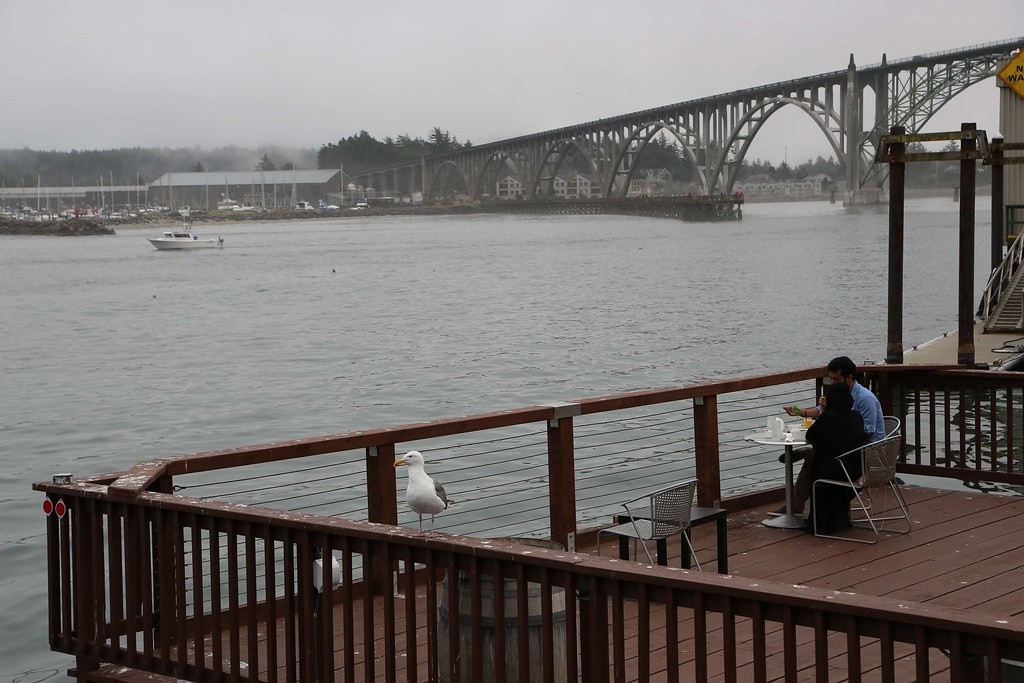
[319,205,339,209]
[357,201,368,206]
[482,192,490,197]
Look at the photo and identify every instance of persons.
[765,356,887,517]
[805,381,865,536]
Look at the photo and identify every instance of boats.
[146,223,224,249]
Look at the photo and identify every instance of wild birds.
[393,451,447,537]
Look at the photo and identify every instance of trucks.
[178,205,201,214]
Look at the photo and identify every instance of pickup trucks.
[233,204,254,212]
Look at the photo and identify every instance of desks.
[744,429,811,528]
[618,504,727,573]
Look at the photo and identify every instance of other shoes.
[779,449,803,463]
[766,505,803,517]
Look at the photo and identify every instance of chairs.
[851,416,909,522]
[597,478,702,571]
[813,435,911,544]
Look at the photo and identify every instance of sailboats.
[217,177,239,209]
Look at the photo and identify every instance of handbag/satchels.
[808,484,853,535]
